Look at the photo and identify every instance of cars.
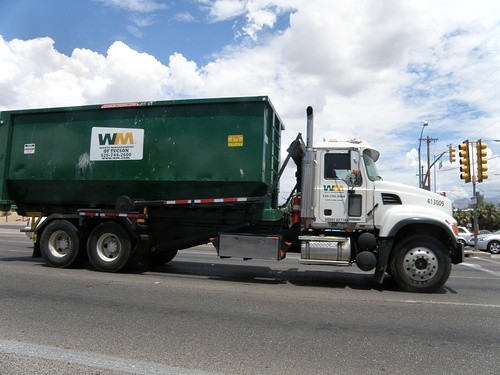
[476,229,500,255]
[458,226,493,247]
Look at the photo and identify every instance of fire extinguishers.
[289,193,301,224]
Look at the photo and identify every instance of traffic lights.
[458,139,472,183]
[449,145,457,165]
[475,138,489,183]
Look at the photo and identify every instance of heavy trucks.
[1,95,465,293]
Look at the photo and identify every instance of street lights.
[418,121,429,188]
